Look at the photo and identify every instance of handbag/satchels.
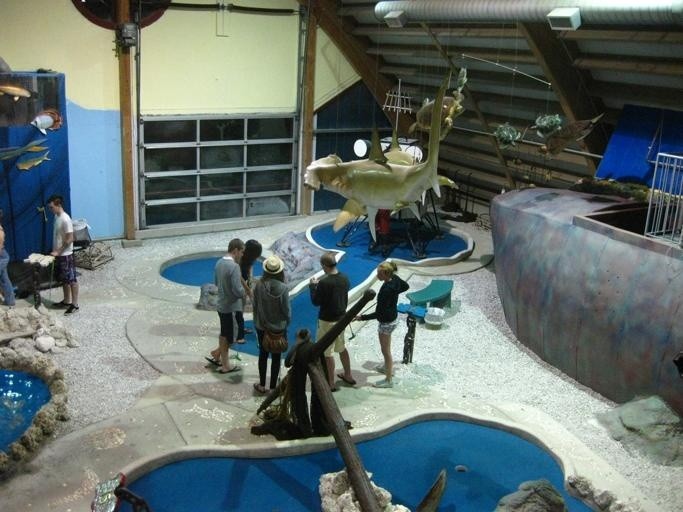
[258,282,288,352]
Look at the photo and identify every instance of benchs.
[406,280,453,324]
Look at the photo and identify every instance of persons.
[236,240,263,344]
[251,255,292,392]
[47,195,80,316]
[0,208,17,306]
[308,253,358,392]
[206,239,244,374]
[353,260,410,389]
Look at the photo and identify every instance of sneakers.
[64,304,79,316]
[52,301,72,309]
[375,366,394,377]
[372,379,394,388]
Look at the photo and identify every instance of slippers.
[254,382,265,392]
[337,373,355,385]
[206,356,222,366]
[330,388,336,392]
[220,365,242,374]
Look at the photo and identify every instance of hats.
[263,255,284,274]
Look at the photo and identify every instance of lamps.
[353,139,371,159]
